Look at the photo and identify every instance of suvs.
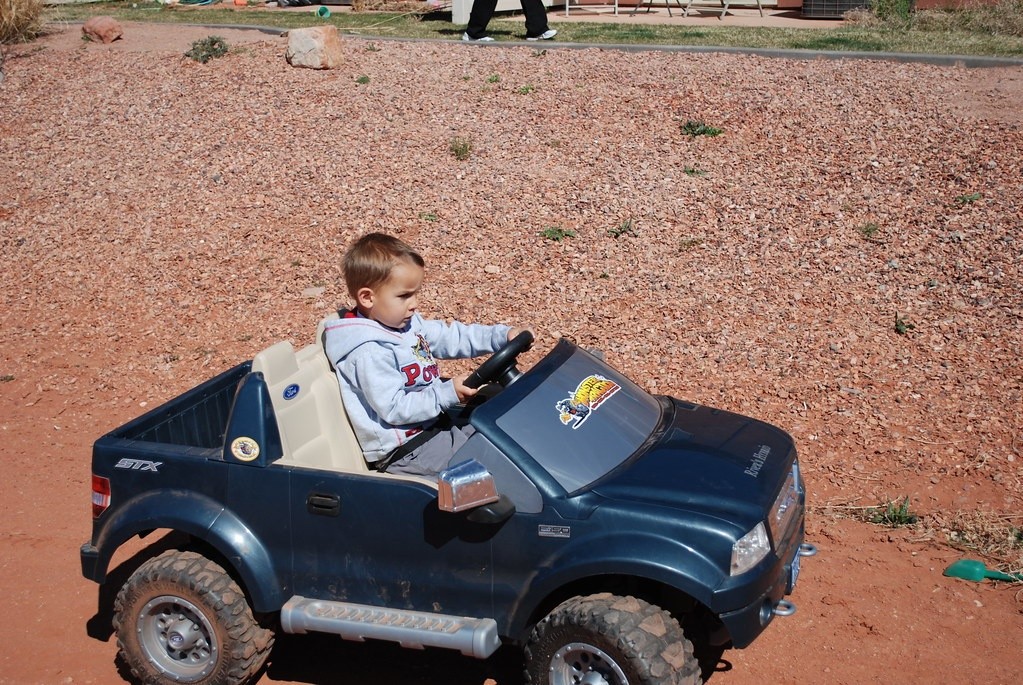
[81,326,807,684]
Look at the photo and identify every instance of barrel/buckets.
[316,7,330,17]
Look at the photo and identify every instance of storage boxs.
[800,0,869,19]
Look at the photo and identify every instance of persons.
[322,233,539,480]
[461,0,557,43]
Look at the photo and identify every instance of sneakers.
[525,29,557,41]
[462,31,494,42]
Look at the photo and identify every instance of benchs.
[252,312,391,476]
